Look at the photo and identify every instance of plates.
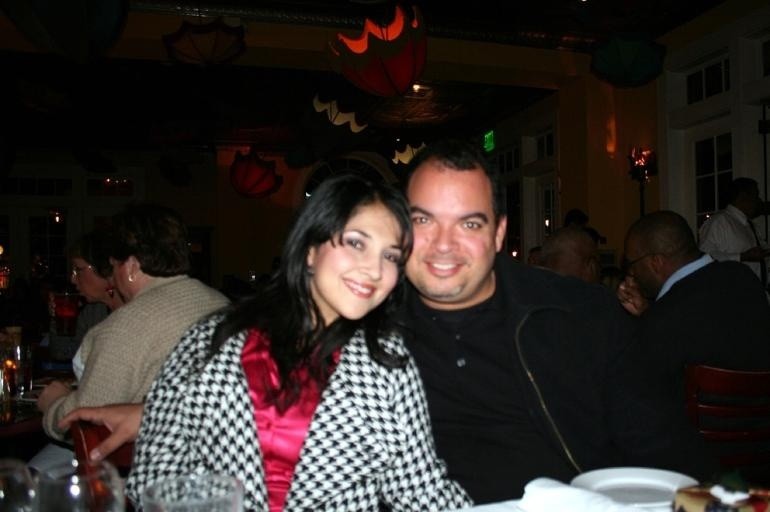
[569,465,700,511]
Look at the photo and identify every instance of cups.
[38,461,125,512]
[0,457,38,512]
[53,289,81,337]
[143,474,245,512]
[0,343,34,406]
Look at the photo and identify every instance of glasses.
[72,266,93,276]
[624,252,666,270]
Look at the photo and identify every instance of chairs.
[682,360,769,491]
